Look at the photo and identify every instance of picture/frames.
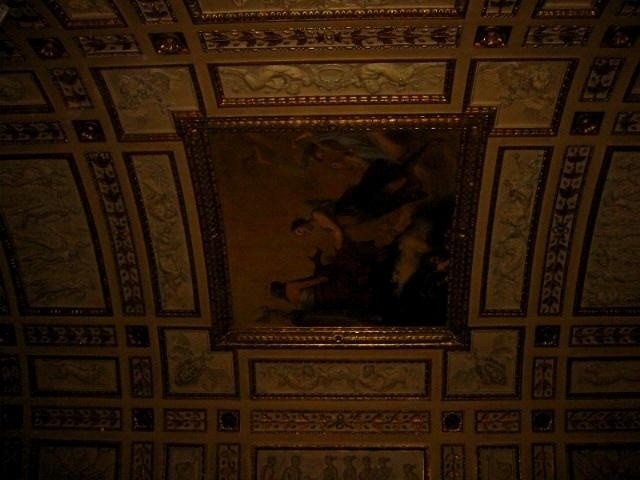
[171,107,496,351]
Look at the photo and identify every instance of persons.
[269,130,456,329]
